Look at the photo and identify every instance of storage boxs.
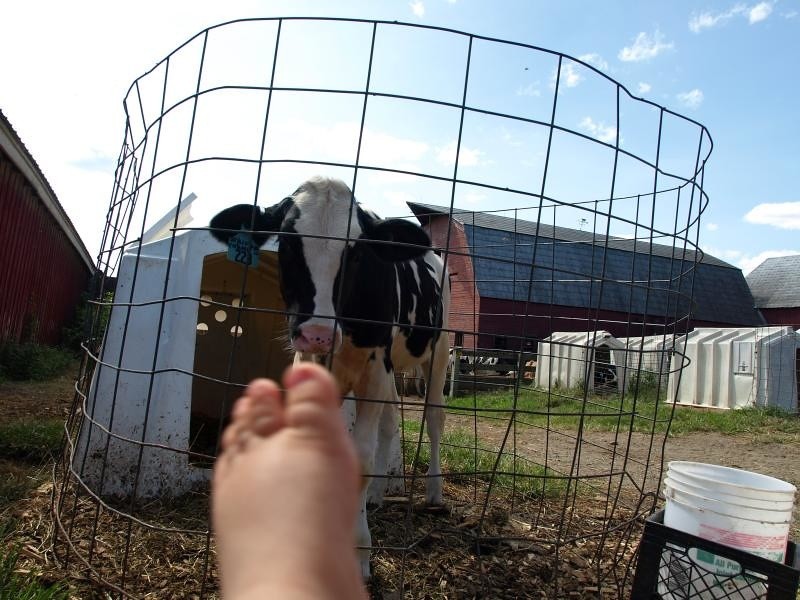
[630,506,800,600]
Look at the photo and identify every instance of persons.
[213,362,369,600]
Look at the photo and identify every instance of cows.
[210,176,449,583]
[401,347,536,398]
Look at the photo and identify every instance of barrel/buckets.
[659,460,796,600]
